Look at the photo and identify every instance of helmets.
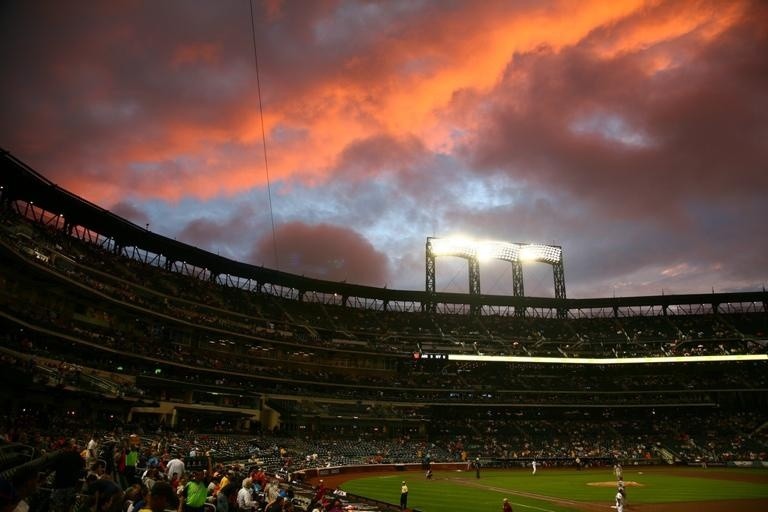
[618,487,625,494]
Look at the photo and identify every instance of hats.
[151,482,177,507]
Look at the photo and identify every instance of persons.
[1,418,343,512]
[475,460,481,479]
[400,481,409,509]
[532,458,537,475]
[503,498,512,512]
[615,464,625,512]
[425,469,433,479]
[1,214,767,467]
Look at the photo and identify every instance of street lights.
[424,235,569,319]
